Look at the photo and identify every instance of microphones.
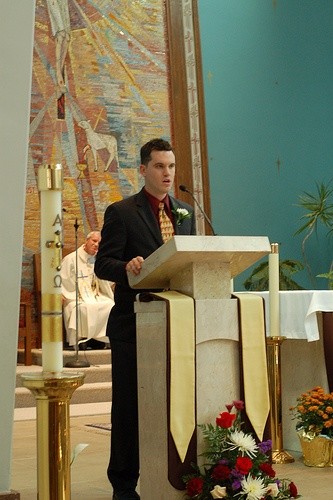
[178,184,216,235]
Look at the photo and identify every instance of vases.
[296,431,333,468]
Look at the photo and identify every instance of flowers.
[289,385,333,437]
[172,207,193,226]
[182,400,301,499]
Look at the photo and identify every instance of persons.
[60,231,115,350]
[92,137,201,500]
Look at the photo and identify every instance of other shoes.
[112,489,140,500]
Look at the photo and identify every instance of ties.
[157,202,175,245]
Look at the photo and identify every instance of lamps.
[20,162,85,500]
